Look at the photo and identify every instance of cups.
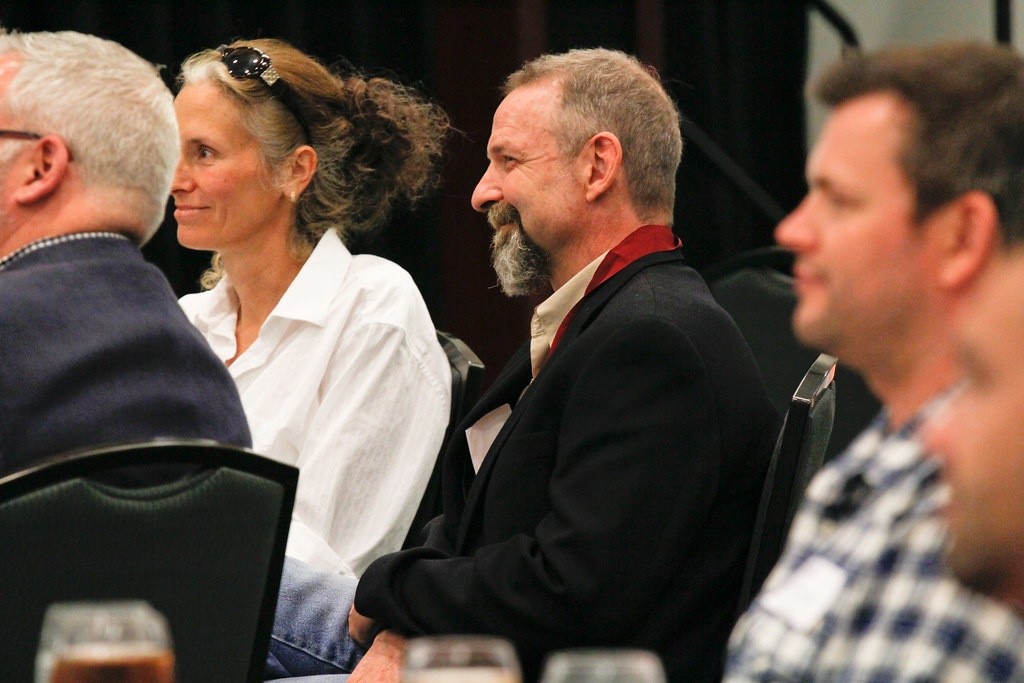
[36,603,176,683]
[402,637,521,683]
[545,651,666,683]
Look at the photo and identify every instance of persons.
[722,46,1024,683]
[0,31,253,475]
[174,38,452,583]
[266,47,770,683]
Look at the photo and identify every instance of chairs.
[0,438,300,683]
[742,352,840,617]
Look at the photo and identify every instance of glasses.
[222,46,313,146]
